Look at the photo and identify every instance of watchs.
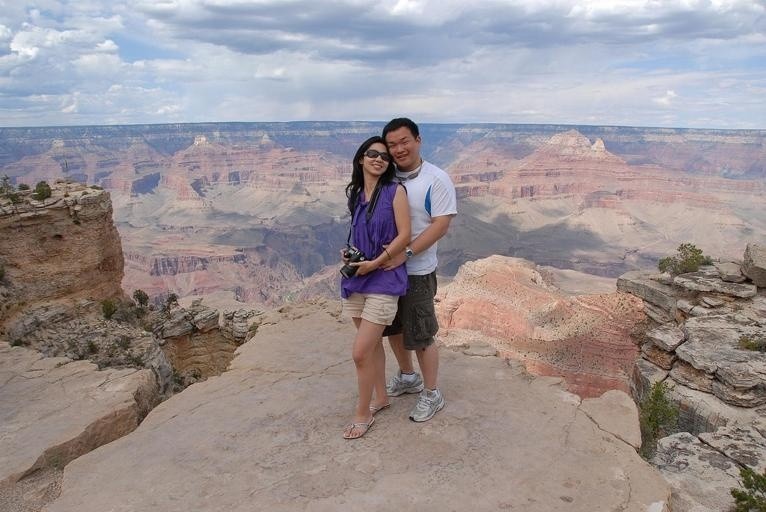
[404,245,414,259]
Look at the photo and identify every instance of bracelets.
[384,249,392,261]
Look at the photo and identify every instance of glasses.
[360,149,391,162]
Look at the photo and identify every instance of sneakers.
[408,386,446,423]
[385,370,424,398]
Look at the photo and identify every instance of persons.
[340,117,457,422]
[338,135,413,439]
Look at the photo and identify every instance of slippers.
[342,416,376,441]
[369,398,391,415]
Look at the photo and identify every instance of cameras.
[340,247,366,280]
